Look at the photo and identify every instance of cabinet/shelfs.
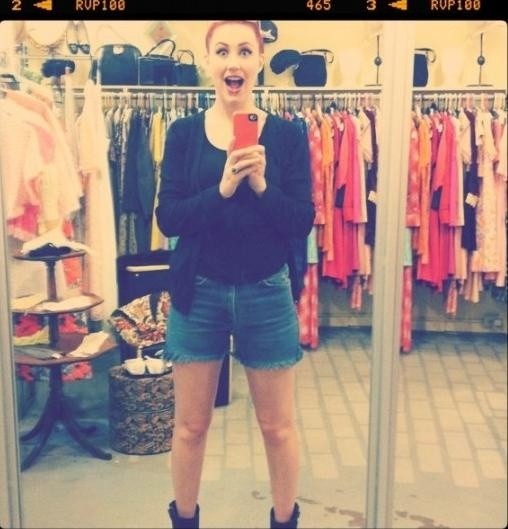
[12,248,112,474]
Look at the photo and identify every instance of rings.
[230,166,238,176]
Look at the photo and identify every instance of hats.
[270,49,301,75]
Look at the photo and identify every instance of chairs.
[114,250,229,408]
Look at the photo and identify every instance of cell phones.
[232,111,259,150]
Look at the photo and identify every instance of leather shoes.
[78,21,91,55]
[67,21,78,54]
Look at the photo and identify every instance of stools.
[109,366,175,455]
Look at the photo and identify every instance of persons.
[156,19,316,529]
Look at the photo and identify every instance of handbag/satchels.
[135,37,175,86]
[293,47,334,87]
[174,48,199,86]
[414,46,437,87]
[99,44,141,86]
